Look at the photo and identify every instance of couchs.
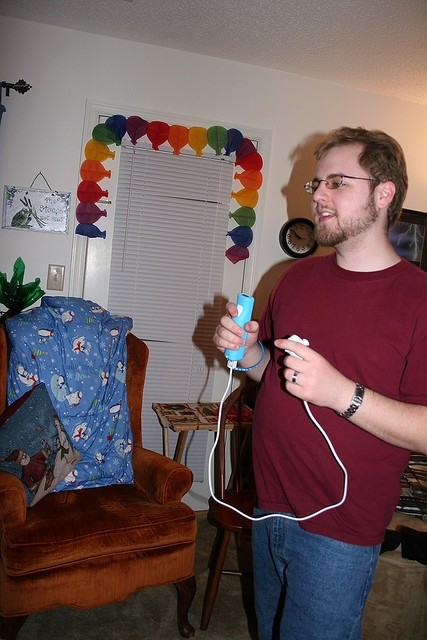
[3,316,198,638]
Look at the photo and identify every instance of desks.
[152,395,256,514]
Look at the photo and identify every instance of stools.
[199,484,277,631]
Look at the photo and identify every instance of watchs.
[339,383,365,420]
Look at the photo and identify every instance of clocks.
[278,217,319,259]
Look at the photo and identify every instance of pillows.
[3,387,86,507]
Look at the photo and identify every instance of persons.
[212,126,427,640]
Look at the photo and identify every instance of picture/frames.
[388,207,427,276]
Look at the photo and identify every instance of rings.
[292,371,298,383]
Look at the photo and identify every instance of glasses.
[303,174,377,195]
